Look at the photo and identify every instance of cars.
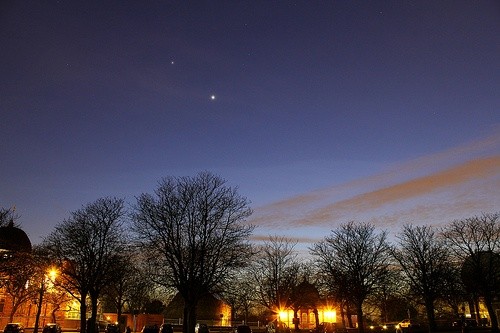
[314,321,344,333]
[3,323,25,333]
[194,323,211,333]
[141,323,173,333]
[104,324,131,333]
[267,321,292,333]
[234,324,252,333]
[398,318,420,328]
[42,323,62,333]
[451,318,478,332]
